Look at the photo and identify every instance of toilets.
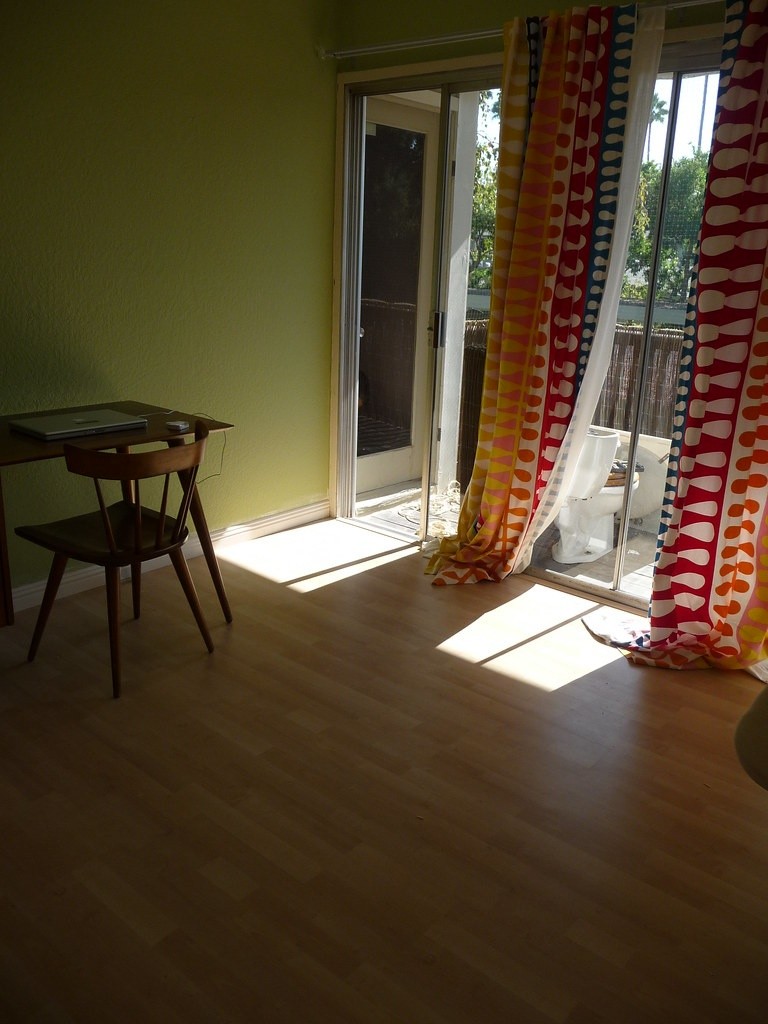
[551,425,640,565]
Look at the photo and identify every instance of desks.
[0,401,234,622]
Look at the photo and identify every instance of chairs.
[13,419,213,696]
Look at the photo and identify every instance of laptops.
[8,409,147,441]
